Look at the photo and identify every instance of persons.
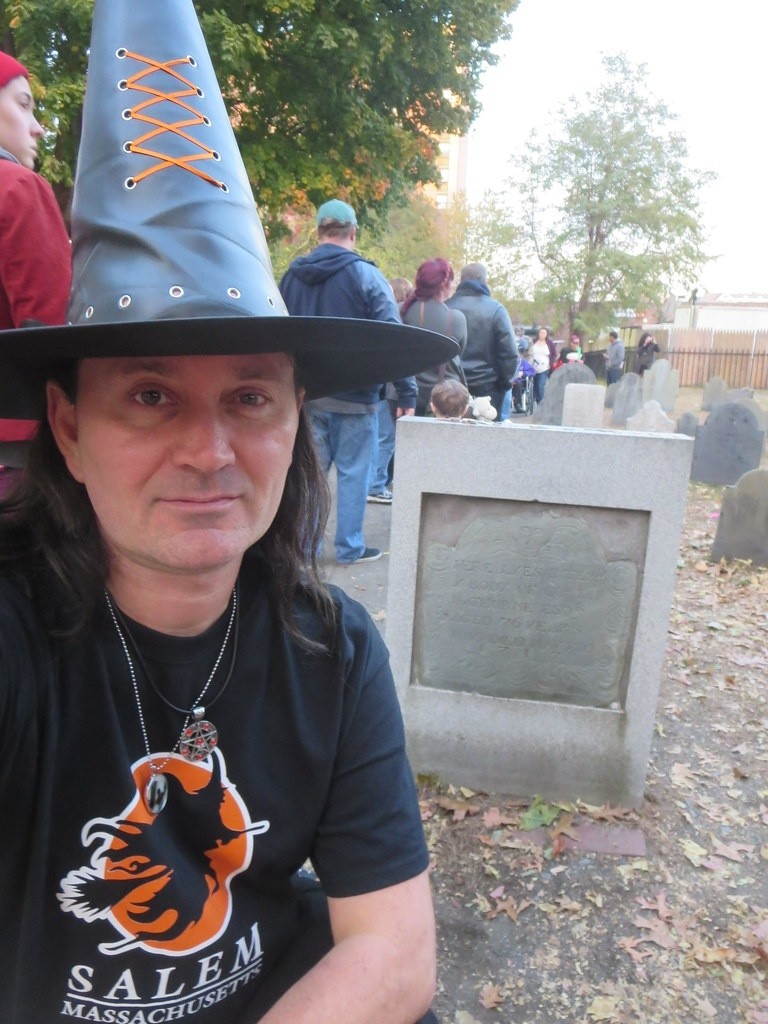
[511,358,536,410]
[400,257,468,416]
[637,332,661,378]
[528,328,556,404]
[0,316,437,1024]
[444,263,521,421]
[0,52,72,494]
[553,335,585,367]
[278,200,418,581]
[367,277,414,504]
[515,326,534,360]
[427,379,476,420]
[604,331,625,388]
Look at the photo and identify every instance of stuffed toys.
[471,394,498,421]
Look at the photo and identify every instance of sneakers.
[367,488,393,502]
[352,546,381,563]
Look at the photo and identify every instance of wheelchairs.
[511,375,534,417]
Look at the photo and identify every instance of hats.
[0,50,28,86]
[315,199,359,228]
[0,0,460,418]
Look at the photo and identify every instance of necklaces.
[108,594,239,762]
[104,588,237,815]
